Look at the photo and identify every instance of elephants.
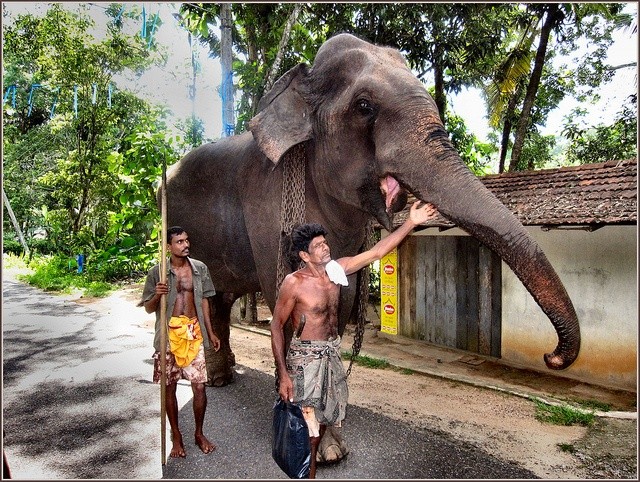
[157,33,580,387]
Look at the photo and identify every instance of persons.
[141,226,222,459]
[269,199,440,479]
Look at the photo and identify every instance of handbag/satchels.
[272,399,311,478]
